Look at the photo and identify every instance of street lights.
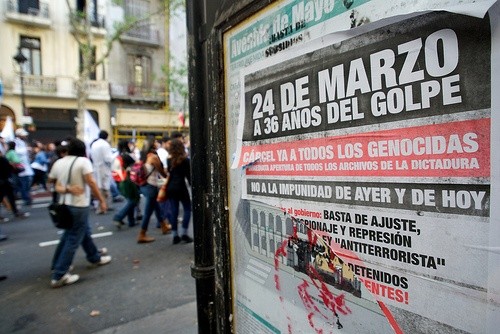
[12,46,29,116]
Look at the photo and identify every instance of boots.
[138,231,155,243]
[160,220,172,234]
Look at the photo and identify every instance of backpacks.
[111,156,126,181]
[130,162,147,185]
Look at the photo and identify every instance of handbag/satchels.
[49,204,73,229]
[156,172,171,201]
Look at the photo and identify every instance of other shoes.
[113,220,121,229]
[136,215,144,220]
[173,236,180,244]
[51,274,80,287]
[182,235,193,243]
[87,255,111,269]
[99,247,108,254]
